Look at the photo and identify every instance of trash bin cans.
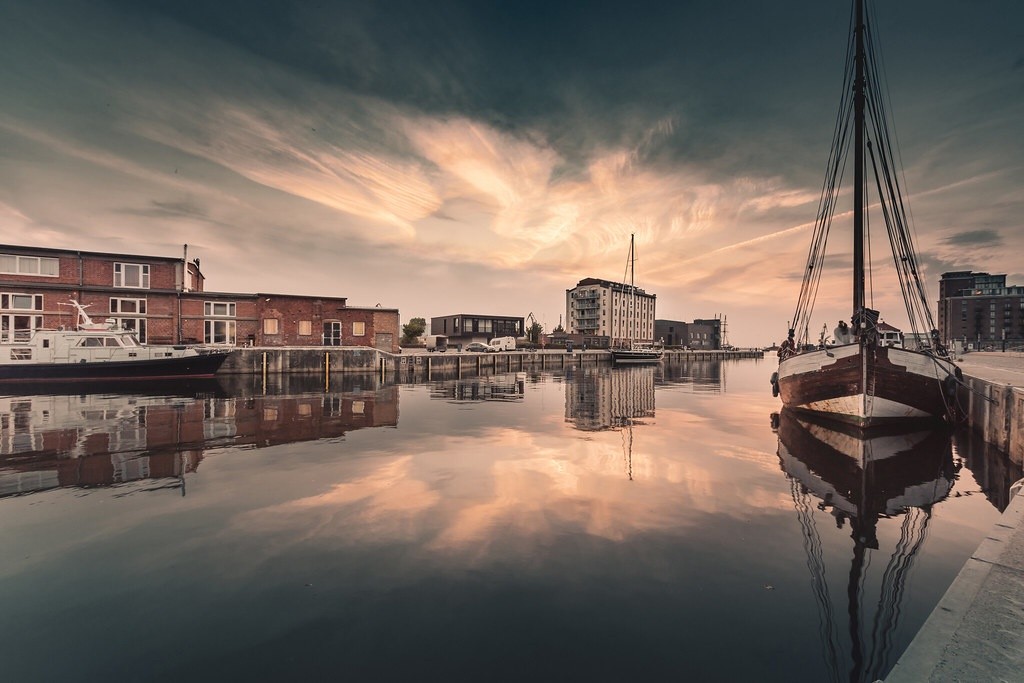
[457,345,461,352]
[567,345,572,352]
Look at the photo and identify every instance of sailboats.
[767,0,964,428]
[608,231,665,364]
[772,401,958,683]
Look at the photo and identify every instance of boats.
[0,298,234,386]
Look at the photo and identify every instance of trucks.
[426,335,448,352]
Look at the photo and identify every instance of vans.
[489,337,516,352]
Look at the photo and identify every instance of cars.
[465,342,495,353]
